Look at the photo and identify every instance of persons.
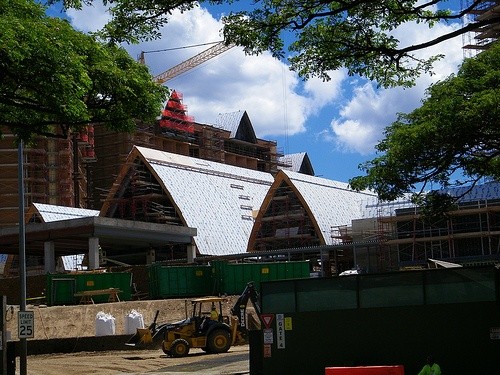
[198,305,219,333]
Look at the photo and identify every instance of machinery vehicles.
[124,296,244,357]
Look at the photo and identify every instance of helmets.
[211,305,216,308]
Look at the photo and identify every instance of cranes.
[139,38,244,85]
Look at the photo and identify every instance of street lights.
[11,119,44,374]
